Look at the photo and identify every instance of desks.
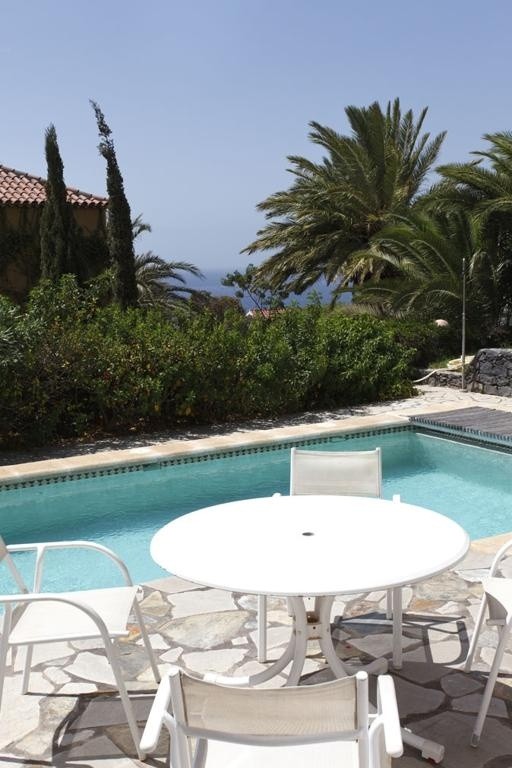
[150,495,471,764]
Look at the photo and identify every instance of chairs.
[139,666,404,768]
[0,536,160,761]
[464,539,512,748]
[258,448,405,670]
